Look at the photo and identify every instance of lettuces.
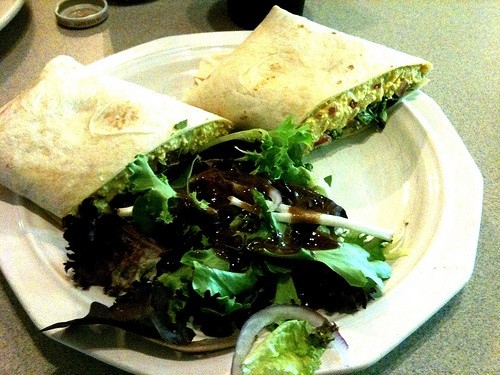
[57,116,407,375]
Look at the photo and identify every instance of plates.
[0,29,484,375]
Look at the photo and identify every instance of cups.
[227,0,305,29]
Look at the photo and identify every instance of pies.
[0,5,433,219]
